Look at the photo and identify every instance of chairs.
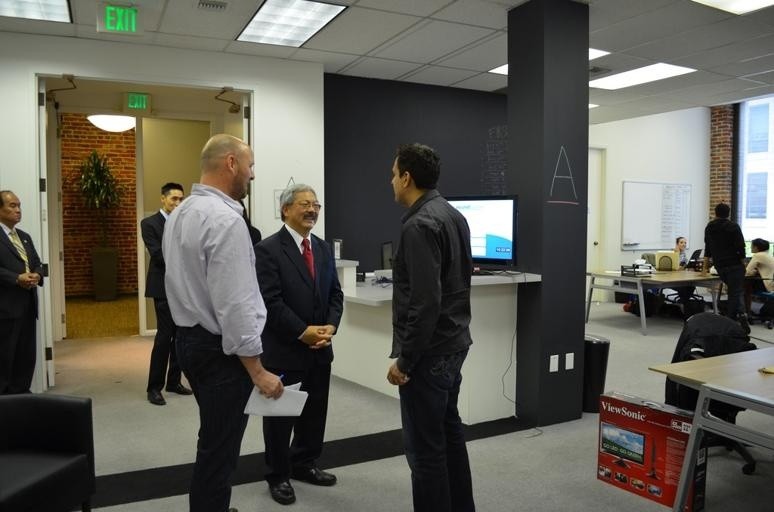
[744,275,774,328]
[0,391,95,512]
[641,252,704,303]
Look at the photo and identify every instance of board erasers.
[623,243,639,246]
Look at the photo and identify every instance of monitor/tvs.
[442,194,518,275]
[690,249,702,261]
[744,240,774,259]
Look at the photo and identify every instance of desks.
[647,344,774,512]
[582,267,725,337]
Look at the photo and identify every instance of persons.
[1,191,45,395]
[670,236,695,300]
[387,142,475,512]
[254,184,344,505]
[140,183,193,406]
[700,203,751,334]
[744,239,774,311]
[161,133,285,512]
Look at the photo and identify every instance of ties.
[6,230,33,290]
[300,238,319,278]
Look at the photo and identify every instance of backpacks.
[664,308,755,436]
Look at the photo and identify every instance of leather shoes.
[289,461,336,484]
[146,390,167,405]
[268,480,296,505]
[166,382,193,395]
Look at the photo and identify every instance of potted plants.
[75,149,122,302]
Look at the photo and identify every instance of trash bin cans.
[583,334,610,413]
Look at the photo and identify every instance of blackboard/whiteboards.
[621,183,691,250]
